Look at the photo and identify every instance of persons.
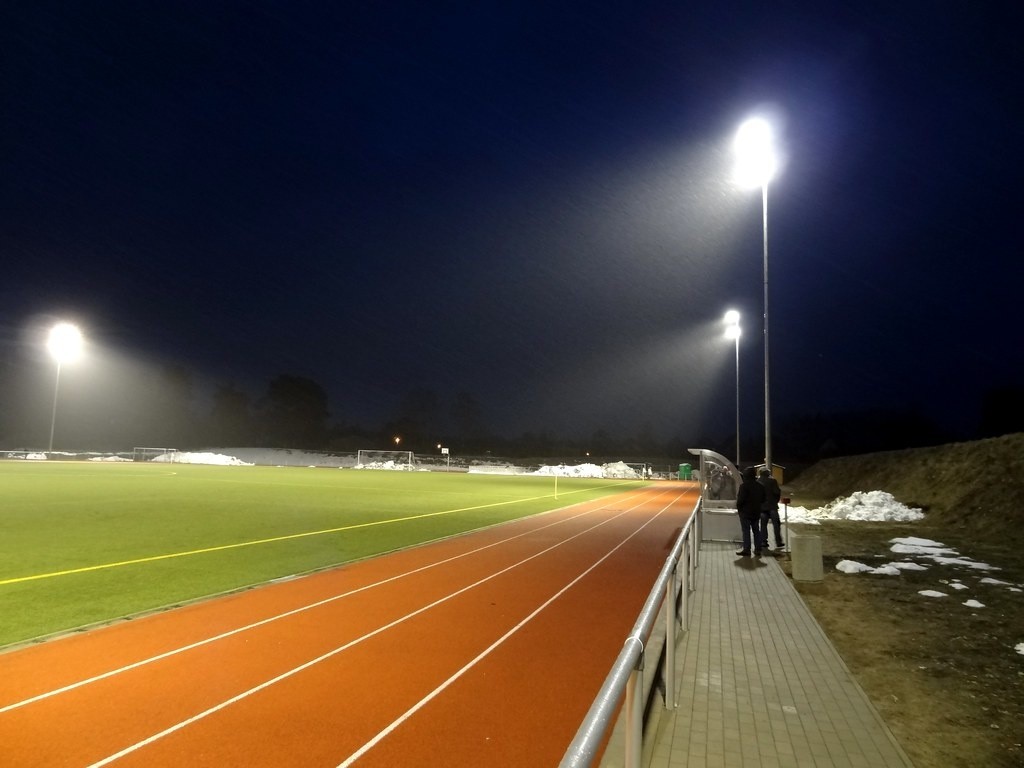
[736,466,765,557]
[647,467,652,480]
[759,464,786,551]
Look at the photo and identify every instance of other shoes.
[735,549,751,556]
[754,549,761,556]
[778,543,785,546]
[760,544,769,547]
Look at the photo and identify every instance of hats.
[759,466,771,473]
[744,469,755,478]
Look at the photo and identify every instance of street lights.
[48,322,68,460]
[725,309,741,467]
[737,115,773,473]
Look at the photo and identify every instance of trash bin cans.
[679,463,692,480]
